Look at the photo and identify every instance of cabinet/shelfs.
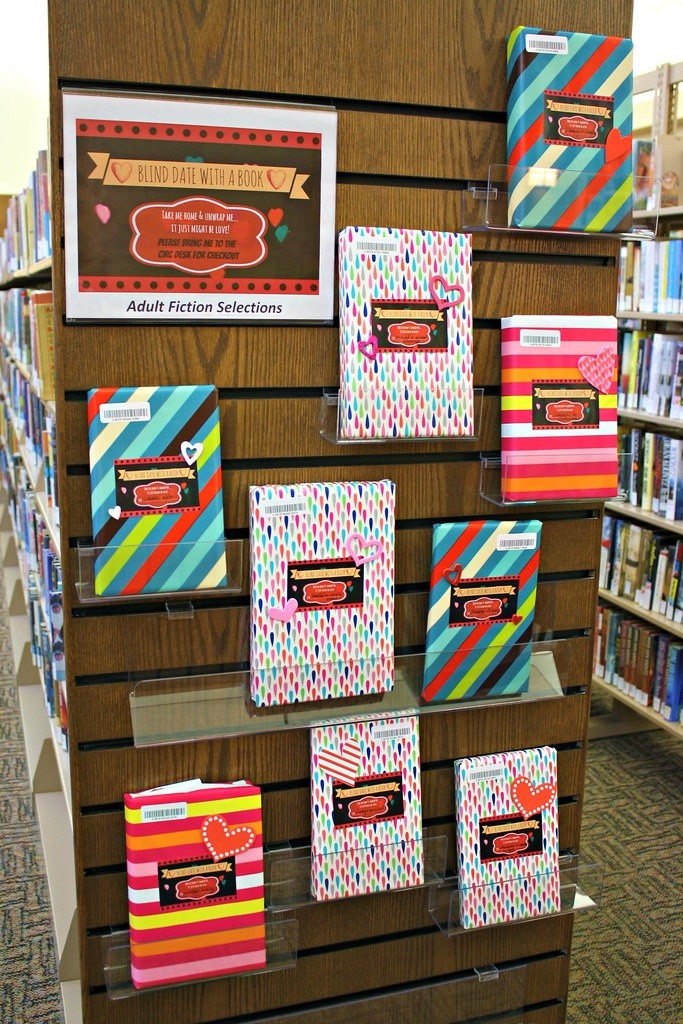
[0,0,637,1024]
[583,61,682,756]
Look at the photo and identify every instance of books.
[338,224,475,437]
[246,479,396,708]
[421,517,544,705]
[454,746,561,930]
[500,314,618,501]
[0,121,70,753]
[123,778,267,990]
[87,384,228,596]
[593,134,683,724]
[310,708,425,901]
[504,26,634,233]
